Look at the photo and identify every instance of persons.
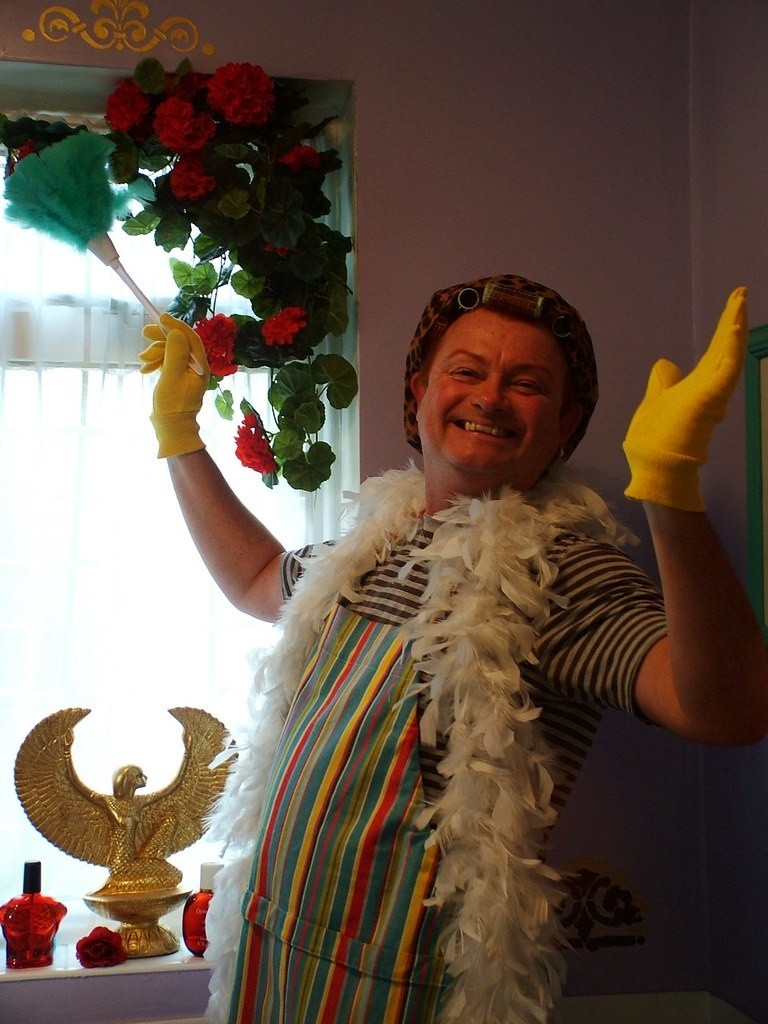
[136,275,768,1024]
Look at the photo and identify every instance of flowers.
[0,55,358,494]
[75,926,126,968]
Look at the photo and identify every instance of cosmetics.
[182,861,228,959]
[0,859,68,970]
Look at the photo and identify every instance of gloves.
[623,285,745,512]
[138,313,210,459]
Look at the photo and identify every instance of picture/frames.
[742,324,768,646]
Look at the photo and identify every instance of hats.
[403,276,598,463]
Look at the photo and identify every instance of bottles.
[0,861,67,969]
